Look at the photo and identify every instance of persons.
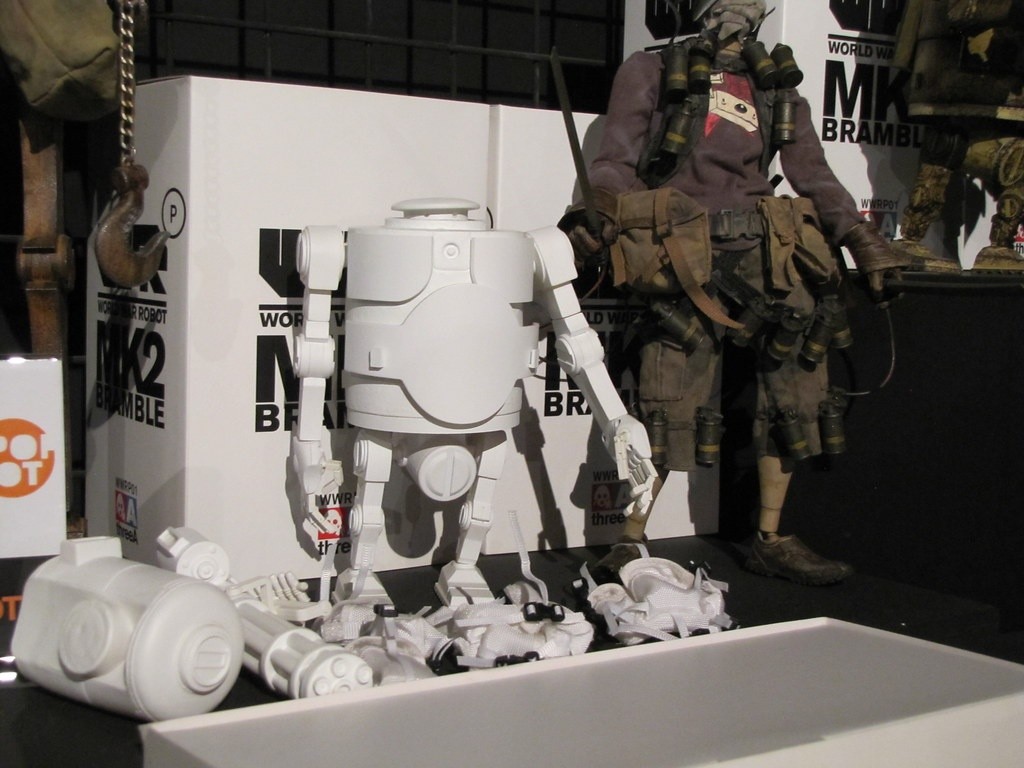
[556,0,905,586]
[889,0,1024,272]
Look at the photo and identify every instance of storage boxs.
[1,358,68,659]
[137,615,1024,768]
[85,0,1024,581]
